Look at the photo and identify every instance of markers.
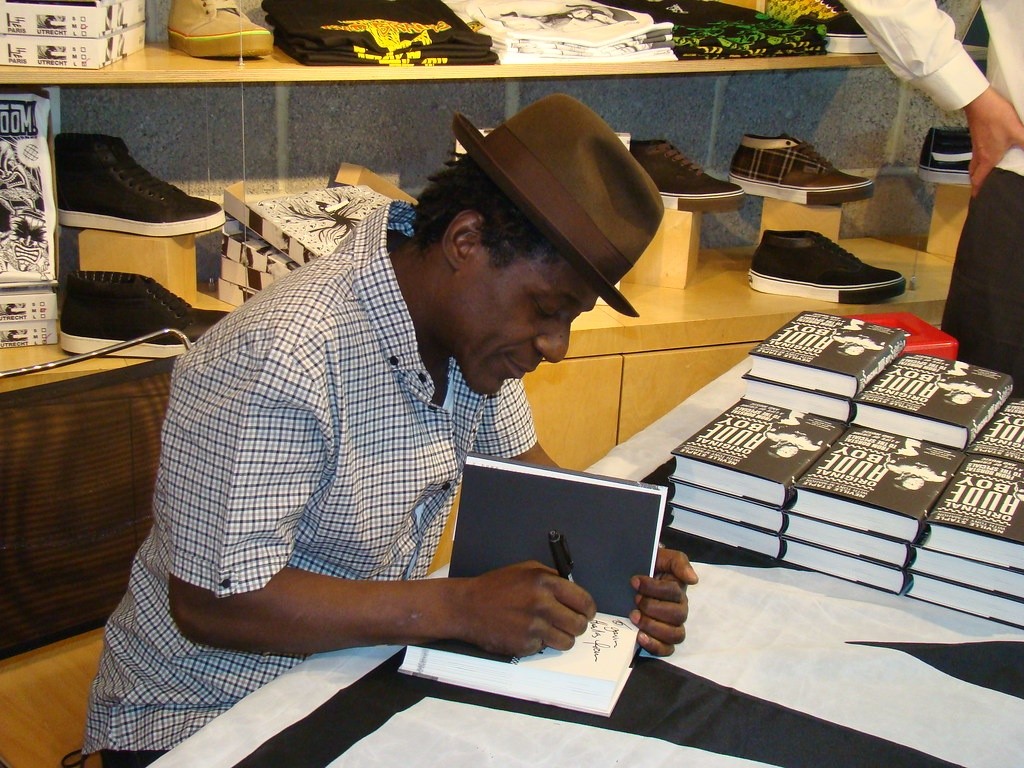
[546,527,573,583]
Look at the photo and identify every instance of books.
[668,311,1024,629]
[398,452,670,719]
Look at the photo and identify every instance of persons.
[840,0,1024,395]
[83,93,700,768]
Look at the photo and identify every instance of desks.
[159,352,1024,768]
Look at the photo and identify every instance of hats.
[451,93,665,318]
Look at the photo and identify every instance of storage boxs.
[227,162,419,265]
[0,85,63,346]
[222,221,299,277]
[0,0,147,71]
[217,279,257,306]
[221,256,278,291]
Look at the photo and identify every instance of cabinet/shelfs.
[0,45,973,567]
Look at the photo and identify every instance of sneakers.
[729,136,876,203]
[748,229,907,303]
[167,0,273,57]
[765,0,877,54]
[626,139,745,212]
[61,268,230,358]
[53,132,225,238]
[917,128,975,185]
